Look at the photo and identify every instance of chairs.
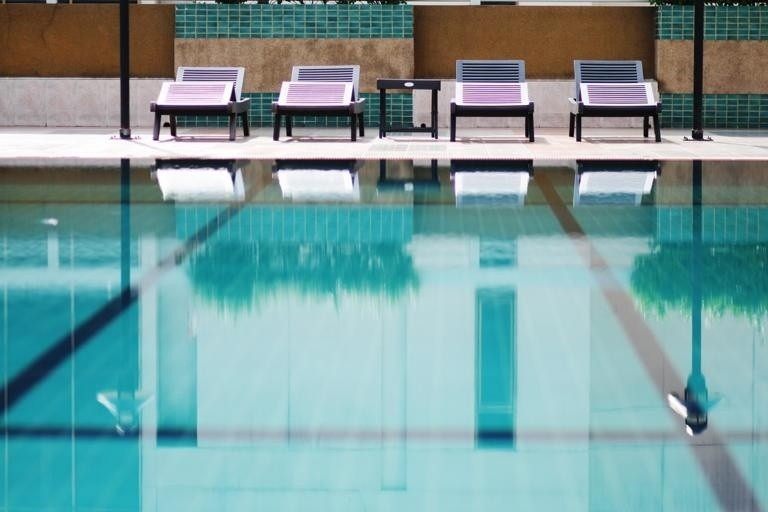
[450,60,534,141]
[272,65,365,141]
[149,66,250,142]
[568,59,663,143]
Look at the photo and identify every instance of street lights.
[85,156,156,443]
[658,159,724,440]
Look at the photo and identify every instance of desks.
[376,78,441,139]
